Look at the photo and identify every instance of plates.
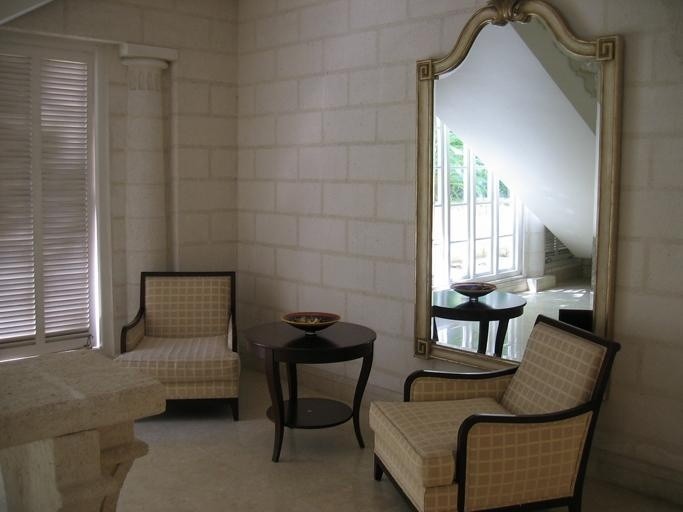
[280,311,342,336]
[450,282,498,300]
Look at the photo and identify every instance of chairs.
[118,269,244,425]
[368,312,620,510]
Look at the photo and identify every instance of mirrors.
[409,0,625,370]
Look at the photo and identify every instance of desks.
[427,289,527,357]
[242,323,376,461]
[0,350,166,512]
